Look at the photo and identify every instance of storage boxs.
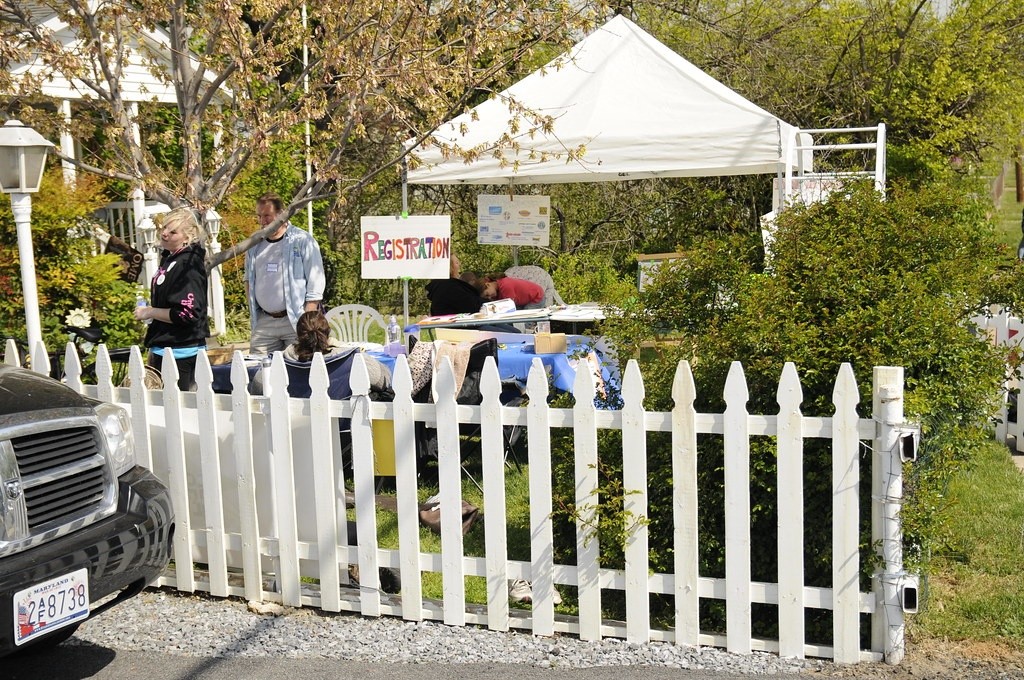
[534,332,566,354]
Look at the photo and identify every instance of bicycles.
[0,324,164,389]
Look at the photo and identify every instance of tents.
[400,16,814,325]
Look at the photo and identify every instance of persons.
[250,311,391,400]
[242,192,326,355]
[460,272,476,285]
[474,272,545,328]
[132,210,211,389]
[425,253,521,333]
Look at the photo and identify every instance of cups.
[534,321,551,334]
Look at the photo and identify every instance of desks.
[209,294,739,495]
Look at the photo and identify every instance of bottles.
[403,324,421,351]
[135,295,153,324]
[385,316,401,347]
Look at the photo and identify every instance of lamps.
[137,214,157,252]
[202,209,222,242]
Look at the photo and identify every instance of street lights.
[0,118,55,376]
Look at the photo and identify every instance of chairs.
[417,338,522,493]
[324,304,388,346]
[80,347,146,388]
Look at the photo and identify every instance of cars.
[0,362,175,663]
